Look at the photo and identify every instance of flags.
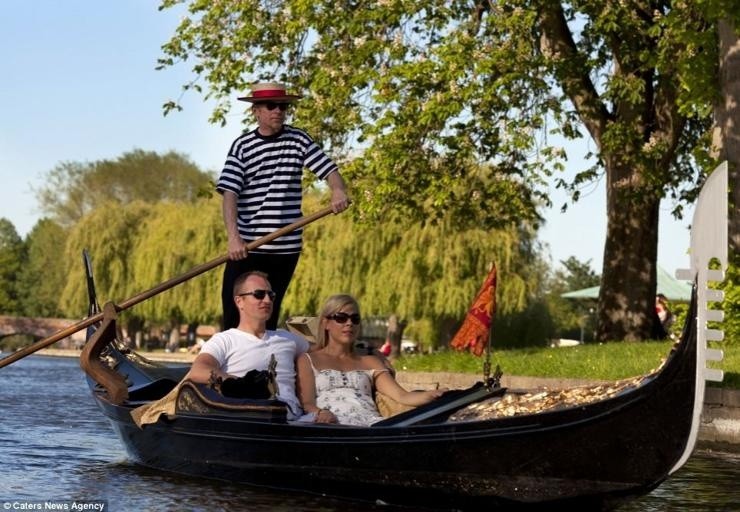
[448,263,497,357]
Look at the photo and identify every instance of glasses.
[326,313,360,324]
[266,102,288,111]
[238,290,276,302]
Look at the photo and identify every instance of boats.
[81,161,728,510]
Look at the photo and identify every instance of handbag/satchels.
[209,361,279,400]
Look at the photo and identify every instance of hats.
[237,83,302,102]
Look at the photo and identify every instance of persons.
[295,294,441,428]
[189,270,340,426]
[655,294,673,335]
[214,83,349,331]
